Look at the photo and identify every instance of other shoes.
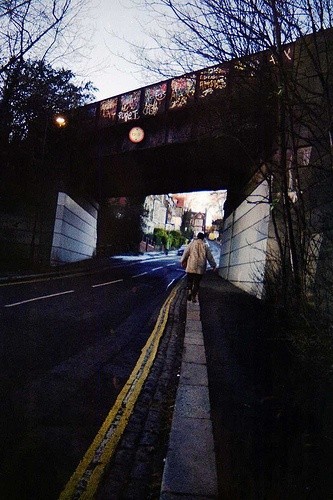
[186,291,198,303]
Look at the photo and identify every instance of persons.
[181,233,217,303]
